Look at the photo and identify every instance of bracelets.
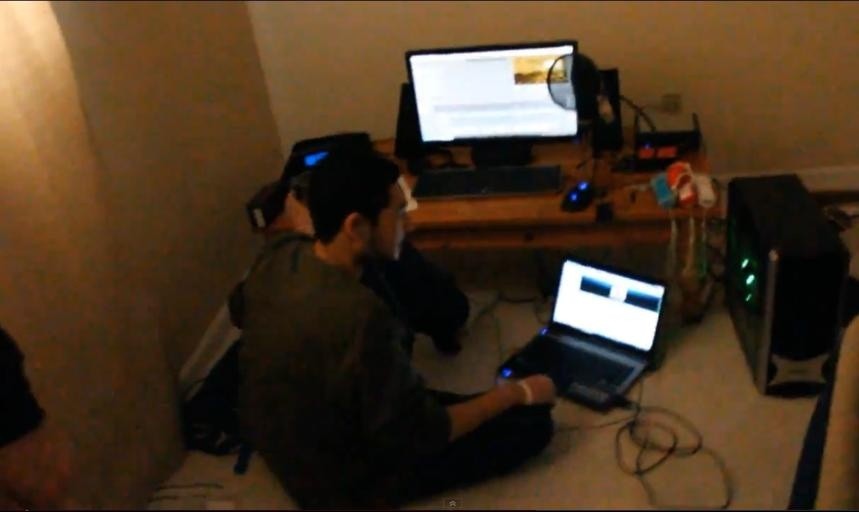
[516,380,534,406]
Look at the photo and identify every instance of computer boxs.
[722,173,850,399]
[279,129,368,195]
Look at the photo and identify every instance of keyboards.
[412,163,562,201]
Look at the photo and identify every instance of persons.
[0,328,73,509]
[225,146,557,511]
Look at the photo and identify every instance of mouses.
[563,183,598,212]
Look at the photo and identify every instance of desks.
[301,133,702,304]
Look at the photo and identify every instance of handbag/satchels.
[174,298,256,475]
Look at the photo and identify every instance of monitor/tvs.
[404,38,579,166]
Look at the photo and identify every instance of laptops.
[493,256,671,415]
